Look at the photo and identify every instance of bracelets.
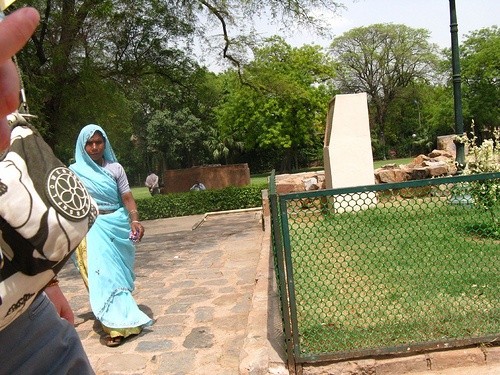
[130,221,141,225]
[129,210,139,217]
[47,279,60,287]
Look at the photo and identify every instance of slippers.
[107,336,121,346]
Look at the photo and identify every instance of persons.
[0,0,95,375]
[145,168,160,196]
[67,123,153,347]
[189,179,207,192]
